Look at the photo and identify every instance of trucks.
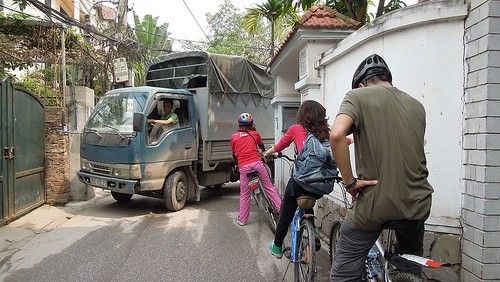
[75,49,276,212]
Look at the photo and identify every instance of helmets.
[239,112,253,123]
[351,54,392,89]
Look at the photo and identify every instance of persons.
[264,101,355,259]
[329,54,434,282]
[148,100,181,140]
[229,113,282,226]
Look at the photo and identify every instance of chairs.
[174,108,187,126]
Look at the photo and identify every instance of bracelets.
[271,145,276,152]
[342,178,358,189]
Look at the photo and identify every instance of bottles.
[367,247,380,277]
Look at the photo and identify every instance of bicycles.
[303,176,441,282]
[265,151,341,282]
[226,155,279,235]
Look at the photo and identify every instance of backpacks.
[292,129,338,196]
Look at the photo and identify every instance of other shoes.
[268,240,283,259]
[236,215,245,225]
[314,228,320,252]
